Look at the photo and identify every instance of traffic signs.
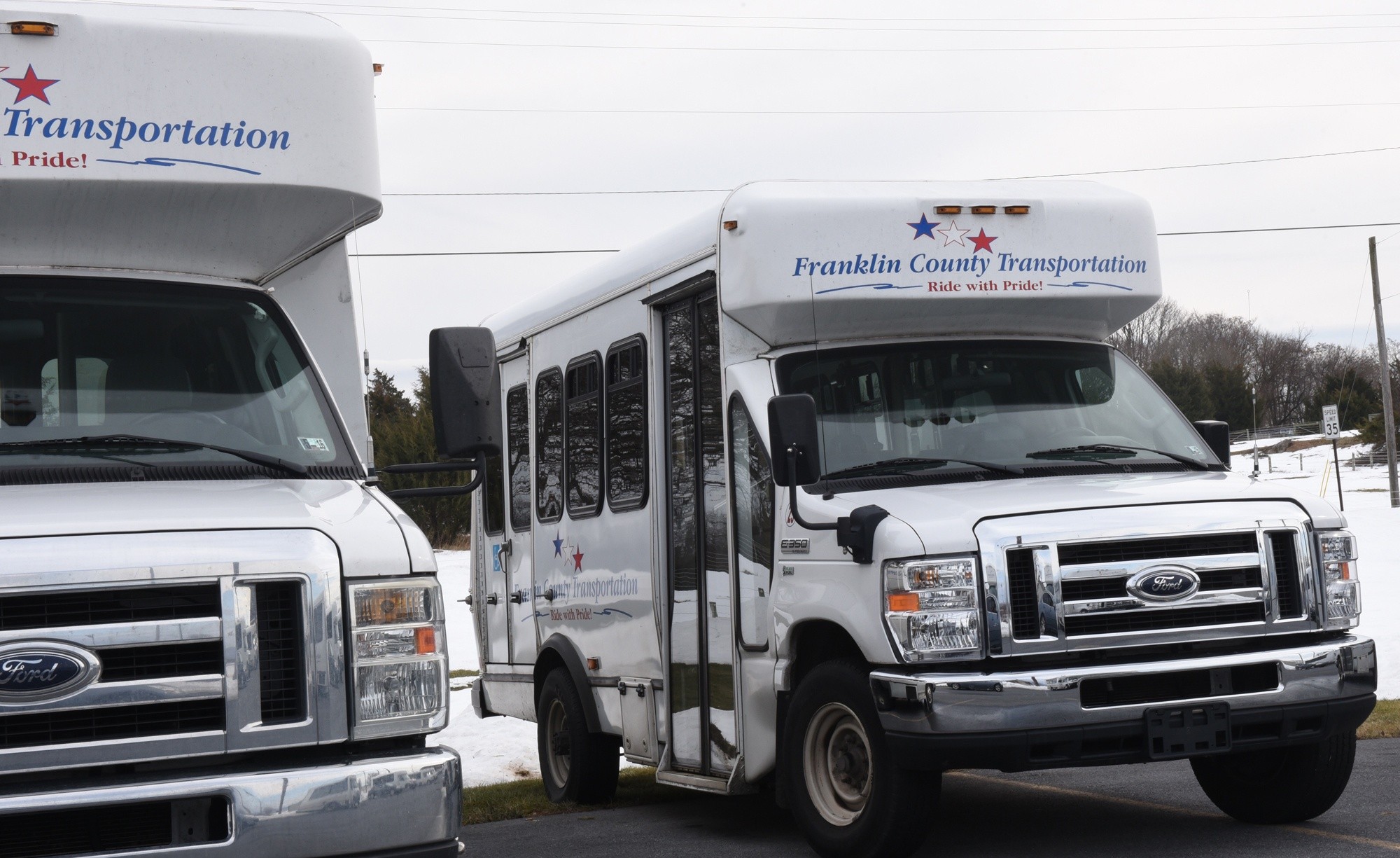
[1321,405,1341,441]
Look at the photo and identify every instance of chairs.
[941,371,1054,445]
[105,327,263,437]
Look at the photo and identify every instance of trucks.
[469,178,1378,856]
[0,7,504,857]
